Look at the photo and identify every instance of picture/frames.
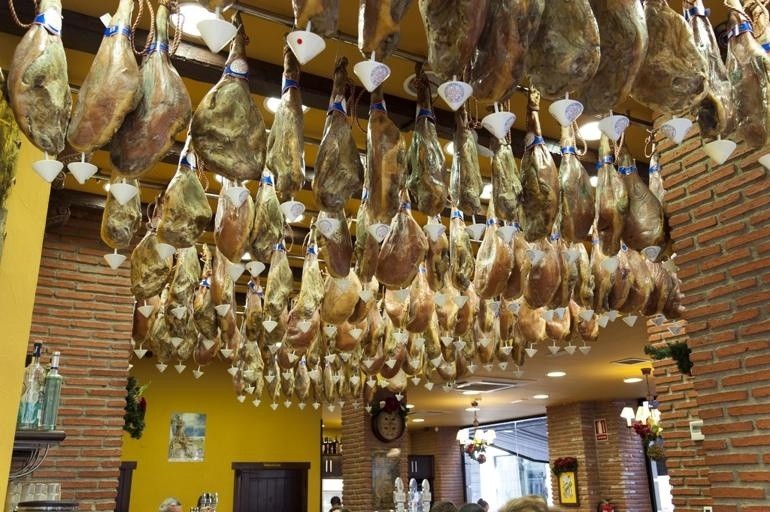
[557,470,580,506]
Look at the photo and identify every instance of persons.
[329,496,342,510]
[503,497,546,511]
[478,499,487,510]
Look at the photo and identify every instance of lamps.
[456,401,496,464]
[620,368,663,441]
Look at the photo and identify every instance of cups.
[6,480,62,511]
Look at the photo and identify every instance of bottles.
[189,491,218,511]
[17,338,62,430]
[322,435,341,455]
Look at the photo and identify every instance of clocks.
[370,386,410,443]
